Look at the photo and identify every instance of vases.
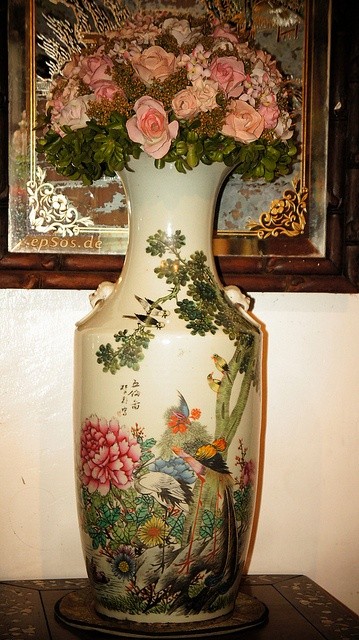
[72,154,264,625]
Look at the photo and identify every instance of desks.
[1,575,358,640]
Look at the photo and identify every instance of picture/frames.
[0,0,359,294]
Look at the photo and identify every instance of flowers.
[32,16,298,187]
